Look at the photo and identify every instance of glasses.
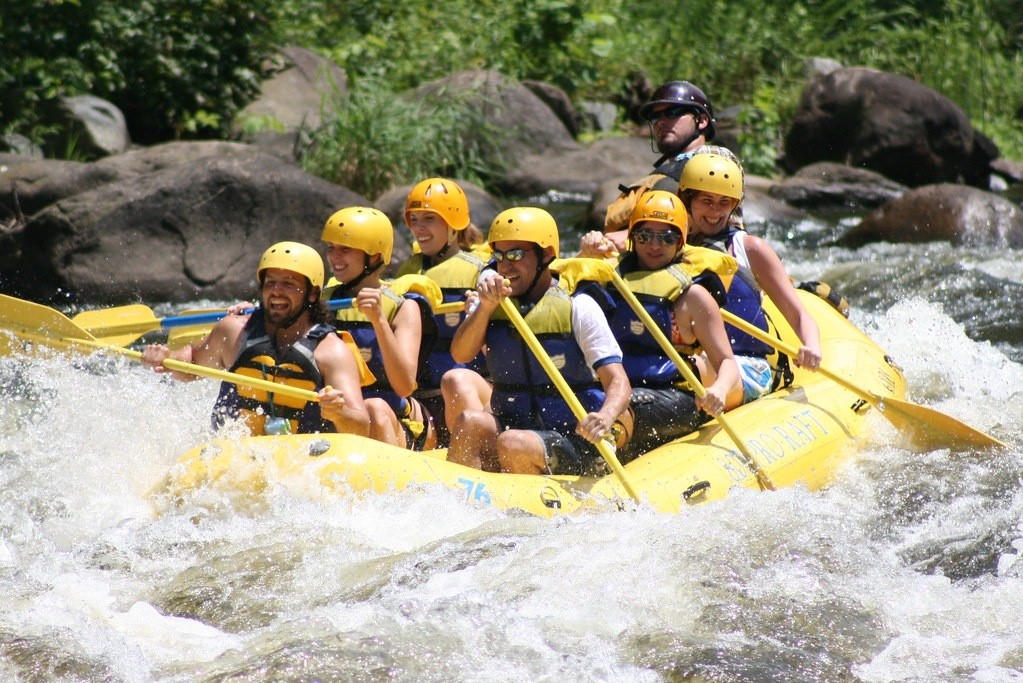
[647,105,698,124]
[633,228,681,247]
[491,246,537,262]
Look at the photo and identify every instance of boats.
[140,278,910,554]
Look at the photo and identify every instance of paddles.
[74,297,359,348]
[603,238,1007,449]
[581,237,777,493]
[573,239,608,258]
[494,277,645,509]
[0,292,334,418]
[165,299,465,348]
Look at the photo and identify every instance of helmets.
[404,177,470,230]
[679,153,742,212]
[257,241,325,294]
[321,206,393,266]
[639,81,717,141]
[487,206,560,261]
[628,191,689,251]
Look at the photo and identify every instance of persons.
[605,80,745,230]
[446,208,634,476]
[606,153,823,410]
[577,190,738,450]
[142,242,370,437]
[227,207,439,449]
[396,179,491,446]
